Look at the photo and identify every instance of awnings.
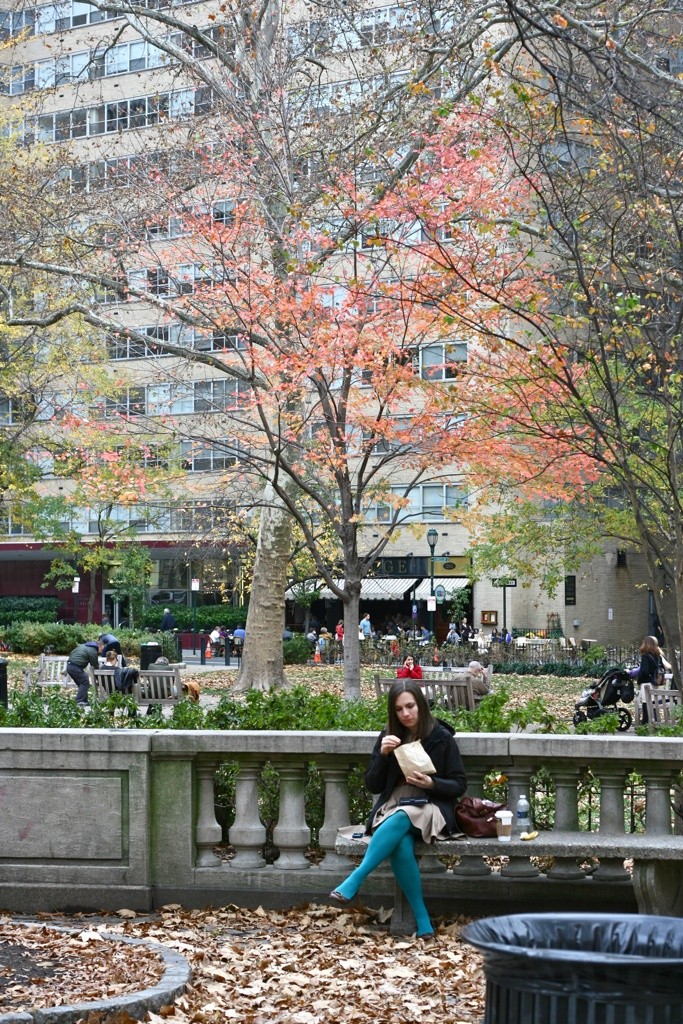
[285,578,417,600]
[410,578,470,600]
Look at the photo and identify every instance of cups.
[495,810,513,842]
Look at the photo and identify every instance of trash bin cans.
[140,644,164,670]
[458,913,682,1024]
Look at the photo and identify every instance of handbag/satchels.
[620,679,634,703]
[454,796,507,838]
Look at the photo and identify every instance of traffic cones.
[314,645,321,663]
[433,647,439,666]
[205,640,212,658]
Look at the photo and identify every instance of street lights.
[426,528,439,662]
[72,525,83,625]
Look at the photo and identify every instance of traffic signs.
[492,578,518,587]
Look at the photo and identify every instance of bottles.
[516,794,530,835]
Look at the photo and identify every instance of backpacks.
[648,653,666,686]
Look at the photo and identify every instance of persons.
[66,608,177,705]
[330,678,468,941]
[209,612,665,725]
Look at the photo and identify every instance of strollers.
[573,668,633,732]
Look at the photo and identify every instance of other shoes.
[329,887,360,905]
[76,701,89,706]
[417,927,437,940]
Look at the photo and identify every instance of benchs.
[644,685,683,726]
[90,666,199,713]
[23,653,93,697]
[336,828,683,938]
[374,674,474,712]
